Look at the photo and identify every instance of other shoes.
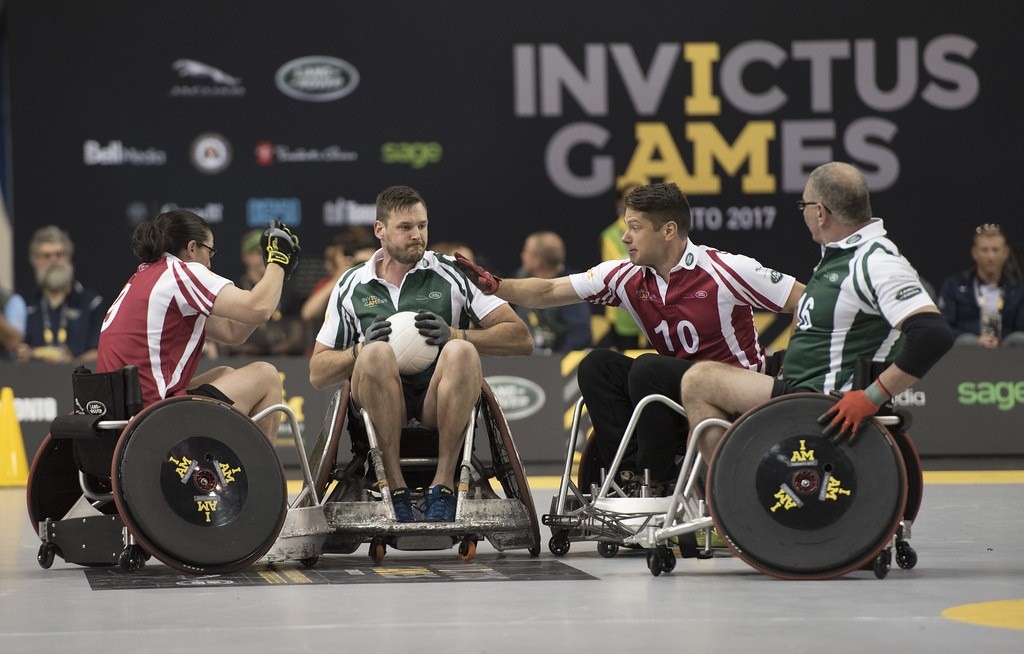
[618,478,678,497]
[668,526,727,549]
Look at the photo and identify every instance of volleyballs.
[388,311,439,376]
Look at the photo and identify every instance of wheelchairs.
[300,376,545,567]
[24,363,337,577]
[641,401,925,580]
[540,395,714,558]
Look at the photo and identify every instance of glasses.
[185,240,218,259]
[796,198,832,216]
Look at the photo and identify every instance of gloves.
[353,314,392,357]
[453,251,503,295]
[415,306,467,347]
[260,220,299,280]
[815,380,894,448]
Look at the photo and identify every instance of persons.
[310,186,533,522]
[513,232,590,349]
[599,184,650,347]
[455,183,807,496]
[681,160,954,470]
[0,226,477,363]
[97,209,299,444]
[938,226,1024,348]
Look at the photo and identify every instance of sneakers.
[425,485,456,522]
[391,488,414,522]
